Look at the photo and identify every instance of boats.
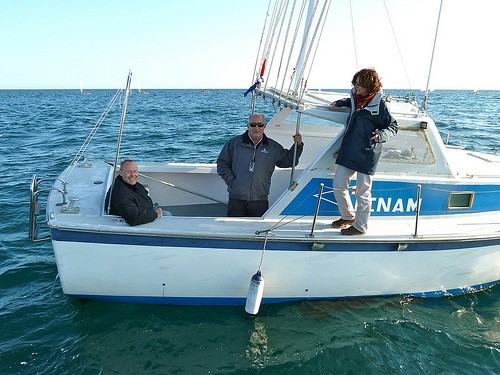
[28,0,499,307]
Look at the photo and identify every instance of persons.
[105,160,172,227]
[216,113,304,217]
[330,68,399,236]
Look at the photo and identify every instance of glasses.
[355,81,362,86]
[249,122,265,127]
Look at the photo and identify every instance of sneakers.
[341,226,363,235]
[332,218,356,228]
